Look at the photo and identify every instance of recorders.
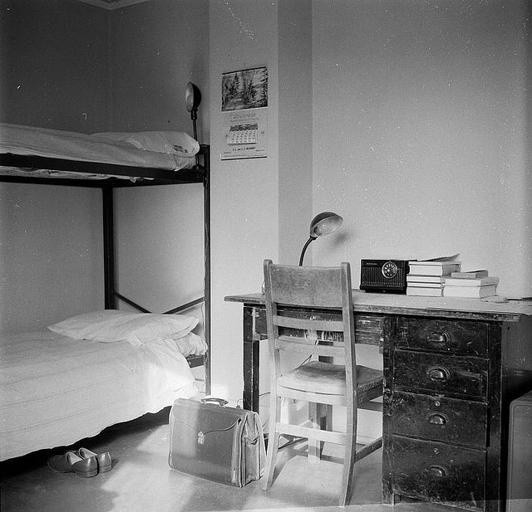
[358,258,418,294]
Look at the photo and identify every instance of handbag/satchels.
[163,394,270,487]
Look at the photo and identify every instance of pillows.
[47,309,200,349]
[90,127,200,156]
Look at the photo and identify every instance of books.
[404,251,500,299]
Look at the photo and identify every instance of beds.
[0,122,211,463]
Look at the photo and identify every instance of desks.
[224,289,532,512]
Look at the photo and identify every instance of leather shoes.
[67,445,114,474]
[46,450,100,479]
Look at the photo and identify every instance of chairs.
[260,259,384,509]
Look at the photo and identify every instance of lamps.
[298,211,344,266]
[183,82,202,141]
